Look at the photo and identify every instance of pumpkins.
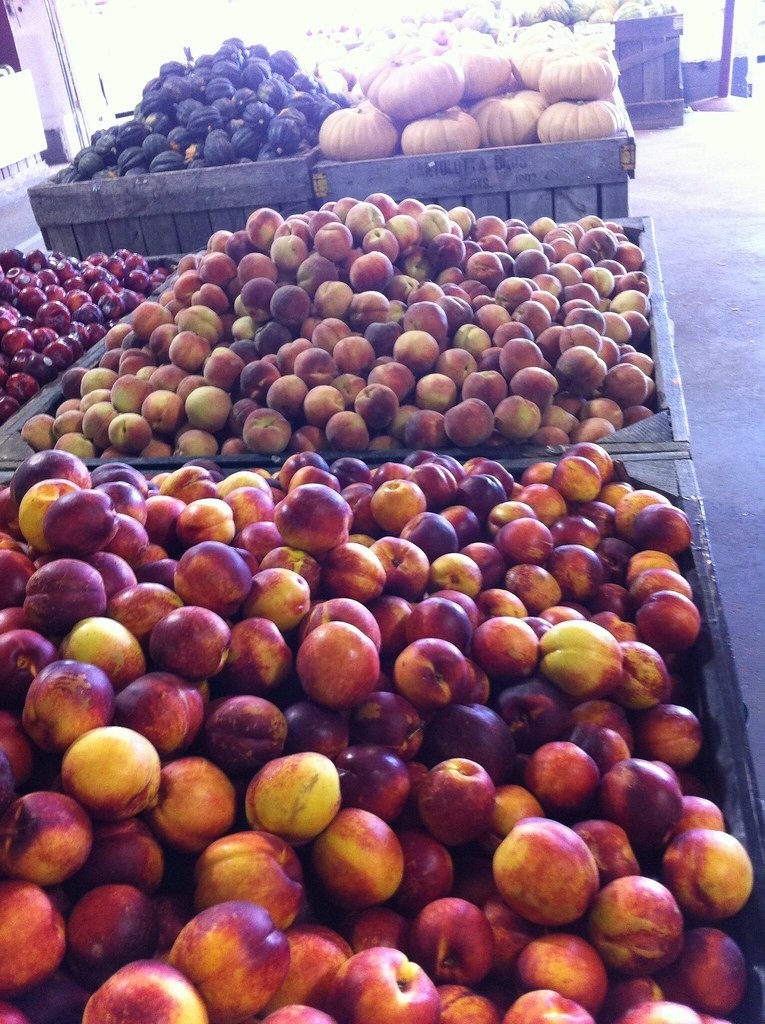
[318,0,678,161]
[49,37,351,185]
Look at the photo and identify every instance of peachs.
[0,193,656,454]
[0,440,753,1024]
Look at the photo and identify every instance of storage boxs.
[610,13,684,132]
[0,253,190,445]
[25,144,324,256]
[0,213,692,472]
[0,451,765,1024]
[309,84,638,227]
